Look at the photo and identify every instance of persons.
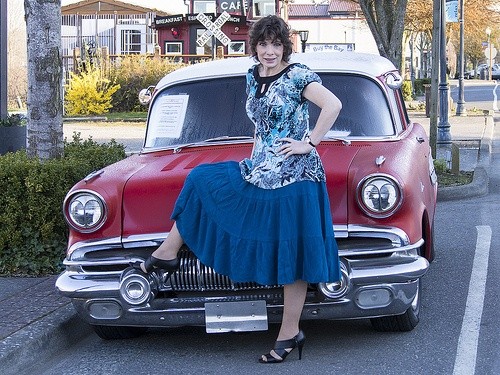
[129,14,342,365]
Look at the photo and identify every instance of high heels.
[128,254,178,283]
[258,330,305,364]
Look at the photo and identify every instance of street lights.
[486,27,492,83]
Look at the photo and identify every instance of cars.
[55,50,438,340]
[464,63,500,81]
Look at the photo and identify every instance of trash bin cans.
[425,84,443,117]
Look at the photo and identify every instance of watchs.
[306,137,316,147]
